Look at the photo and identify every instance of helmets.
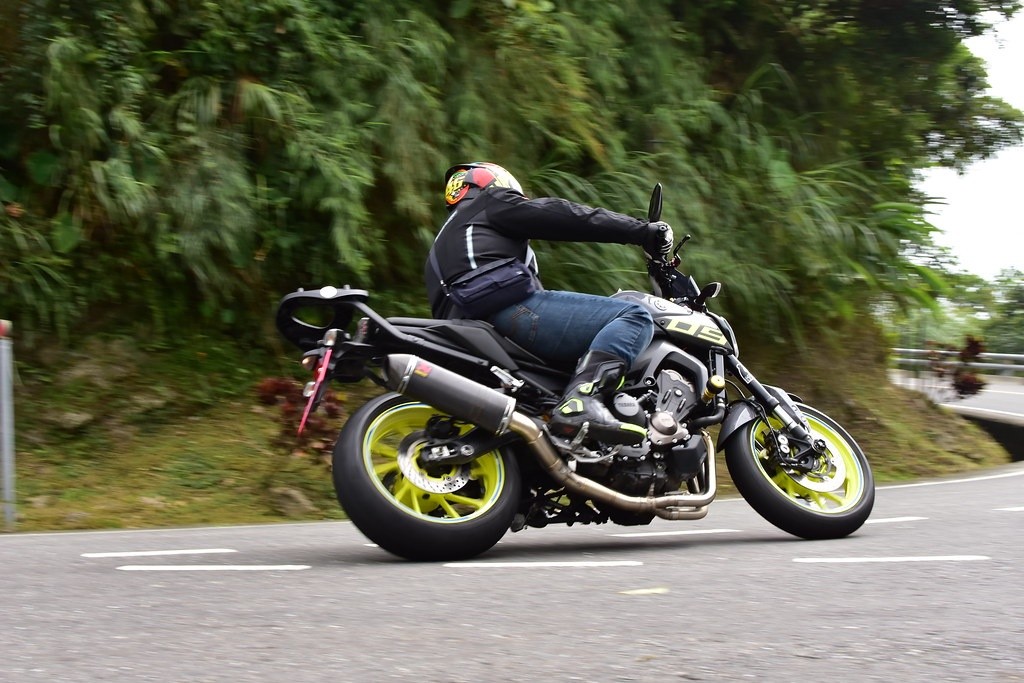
[445,162,526,213]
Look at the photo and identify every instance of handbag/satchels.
[449,257,535,318]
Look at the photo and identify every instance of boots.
[551,351,646,446]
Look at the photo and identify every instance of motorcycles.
[274,183,876,563]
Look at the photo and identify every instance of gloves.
[629,221,673,254]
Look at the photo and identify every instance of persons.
[423,161,674,447]
